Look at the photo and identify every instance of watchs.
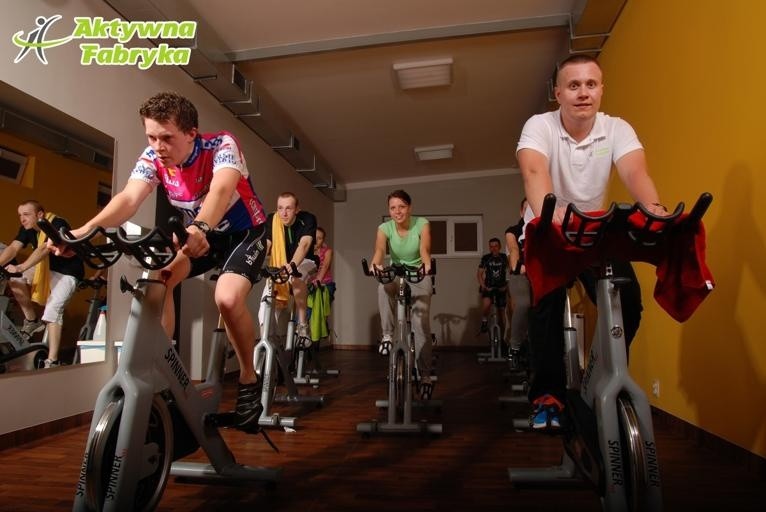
[186,220,210,233]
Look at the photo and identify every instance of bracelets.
[652,203,669,212]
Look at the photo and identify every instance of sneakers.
[42,357,62,370]
[232,371,263,430]
[509,347,521,372]
[17,317,45,342]
[480,318,489,333]
[419,382,435,401]
[532,402,561,429]
[294,322,313,351]
[377,333,392,357]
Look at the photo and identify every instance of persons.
[258,191,319,349]
[515,54,669,437]
[504,197,532,371]
[294,226,336,345]
[368,189,434,403]
[47,91,267,429]
[477,238,515,347]
[0,198,85,367]
